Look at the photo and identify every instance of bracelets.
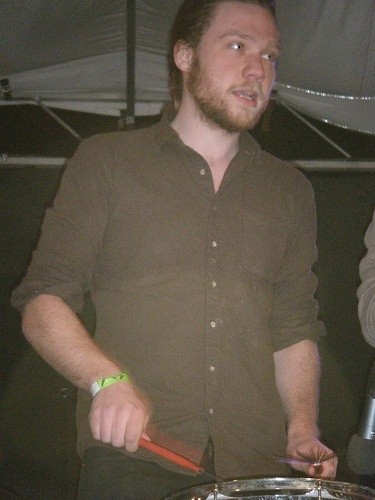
[89,372,131,398]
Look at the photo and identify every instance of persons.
[10,0,339,500]
[357,214,375,351]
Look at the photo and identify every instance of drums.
[162,475,375,500]
